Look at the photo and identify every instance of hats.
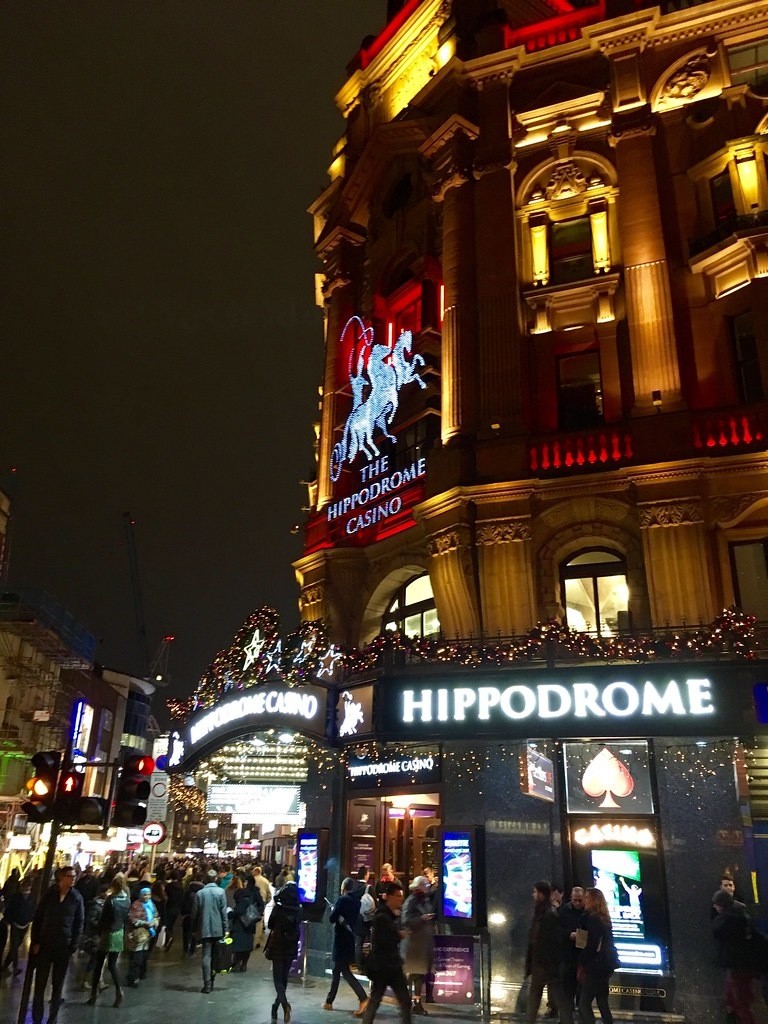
[712,889,732,906]
[140,872,151,882]
[114,872,125,879]
[140,888,151,895]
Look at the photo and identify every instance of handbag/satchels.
[156,926,166,947]
[239,897,261,928]
[270,927,290,949]
[127,926,150,948]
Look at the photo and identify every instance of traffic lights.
[54,770,107,824]
[21,751,60,821]
[112,752,155,827]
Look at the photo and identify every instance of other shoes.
[200,982,214,993]
[354,997,372,1017]
[87,996,96,1005]
[99,984,110,993]
[412,999,427,1016]
[241,960,247,972]
[163,937,174,953]
[321,1003,333,1010]
[14,969,22,977]
[272,1004,278,1019]
[113,991,124,1008]
[78,982,92,990]
[358,969,365,975]
[541,1002,558,1019]
[284,1003,292,1023]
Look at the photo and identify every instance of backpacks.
[359,884,377,922]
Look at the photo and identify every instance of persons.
[710,877,759,1024]
[523,881,622,1024]
[0,854,440,1022]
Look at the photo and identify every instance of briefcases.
[211,933,235,976]
